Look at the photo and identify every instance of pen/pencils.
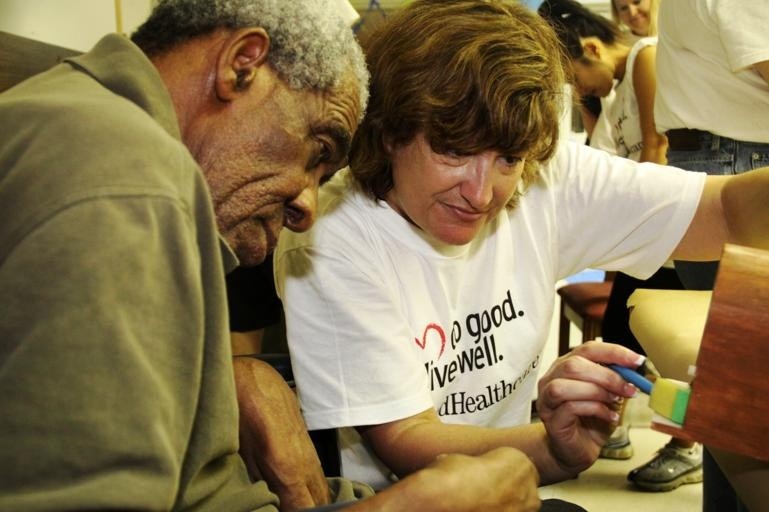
[603,361,654,395]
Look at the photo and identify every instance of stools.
[556,279,614,357]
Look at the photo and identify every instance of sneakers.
[626,440,705,491]
[598,424,634,459]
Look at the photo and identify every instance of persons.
[0,0,540,512]
[272,0,769,512]
[539,0,769,490]
